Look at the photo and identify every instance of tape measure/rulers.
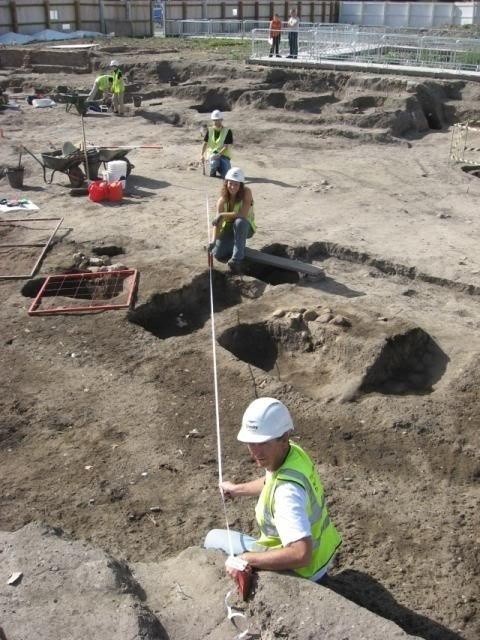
[201,157,257,573]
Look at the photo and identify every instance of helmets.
[237,397,294,443]
[110,60,119,67]
[224,167,244,182]
[211,110,223,120]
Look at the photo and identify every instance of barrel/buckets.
[108,160,127,180]
[132,96,141,107]
[6,167,24,188]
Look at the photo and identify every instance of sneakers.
[228,258,247,266]
[269,54,297,59]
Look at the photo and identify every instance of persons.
[85,75,113,101]
[206,168,256,265]
[109,60,126,115]
[268,13,282,58]
[287,8,300,59]
[203,395,345,581]
[200,110,233,179]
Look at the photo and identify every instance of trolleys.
[23,140,137,188]
[57,93,90,115]
